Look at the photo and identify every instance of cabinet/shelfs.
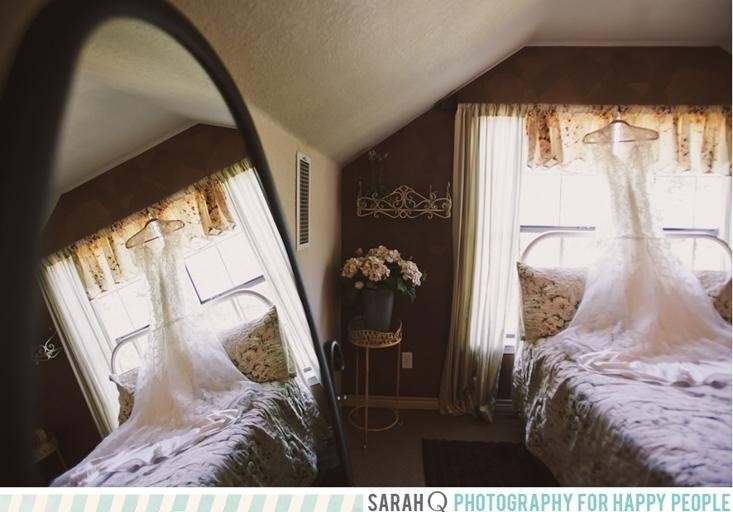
[347,318,403,449]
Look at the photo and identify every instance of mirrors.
[0,0,349,489]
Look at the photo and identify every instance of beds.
[510,225,733,488]
[42,290,328,486]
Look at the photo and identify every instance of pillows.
[513,258,732,346]
[109,303,287,427]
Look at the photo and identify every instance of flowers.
[339,242,422,304]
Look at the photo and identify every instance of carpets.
[420,436,552,487]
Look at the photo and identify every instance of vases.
[360,287,395,335]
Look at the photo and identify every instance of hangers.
[581,119,660,146]
[123,218,185,250]
